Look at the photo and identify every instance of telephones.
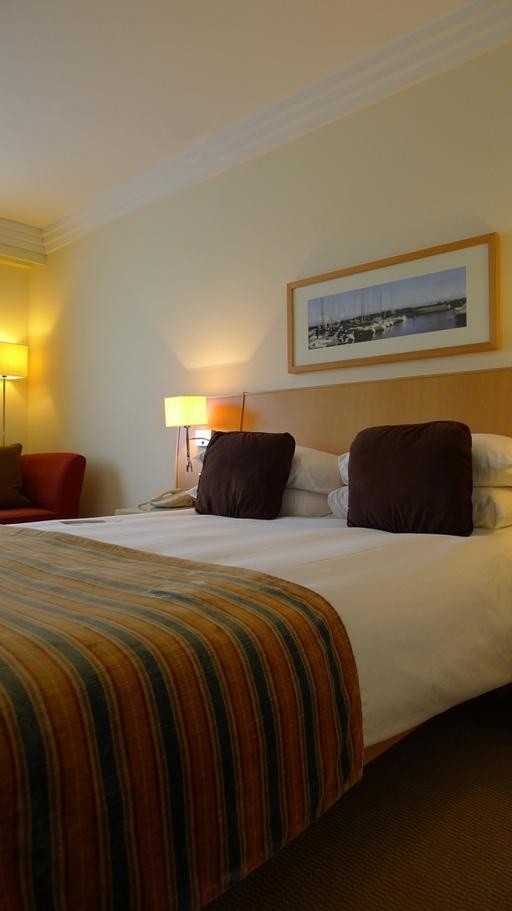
[150,487,193,507]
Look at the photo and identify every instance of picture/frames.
[286,233,500,374]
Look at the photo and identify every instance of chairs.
[0,453,86,524]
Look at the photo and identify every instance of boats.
[454,303,465,314]
[415,304,451,315]
[309,315,406,350]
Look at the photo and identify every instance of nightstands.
[115,503,195,515]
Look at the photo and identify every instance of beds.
[1,368,511,911]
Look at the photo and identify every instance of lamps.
[0,341,28,380]
[164,396,207,473]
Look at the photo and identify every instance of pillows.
[0,443,31,509]
[194,428,296,521]
[345,419,473,537]
